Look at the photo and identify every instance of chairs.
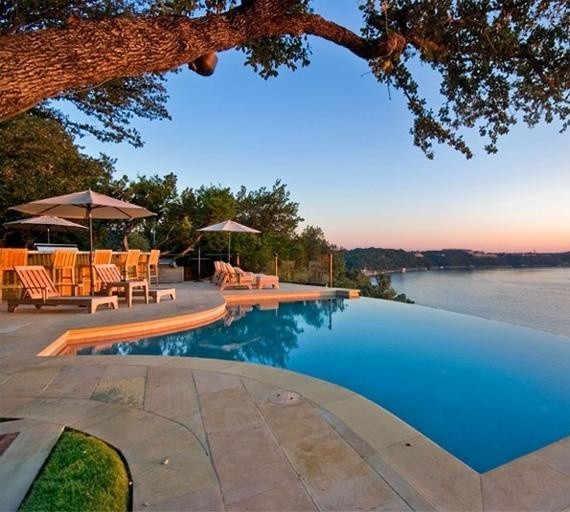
[0,248,175,313]
[212,261,279,291]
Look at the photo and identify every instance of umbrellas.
[2,214,90,244]
[196,219,262,263]
[8,187,158,297]
